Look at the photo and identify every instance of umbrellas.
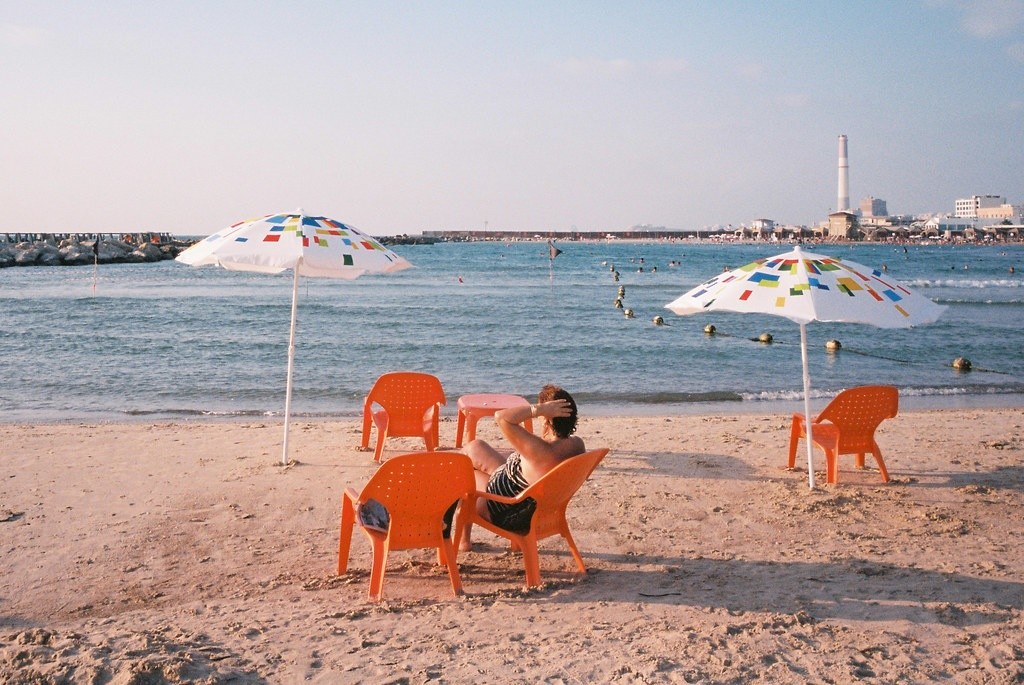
[664,246,950,489]
[176,207,419,467]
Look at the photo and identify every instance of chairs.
[335,450,478,602]
[786,383,904,487]
[360,370,448,466]
[453,444,612,593]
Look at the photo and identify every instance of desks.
[454,393,535,449]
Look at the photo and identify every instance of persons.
[431,384,587,544]
